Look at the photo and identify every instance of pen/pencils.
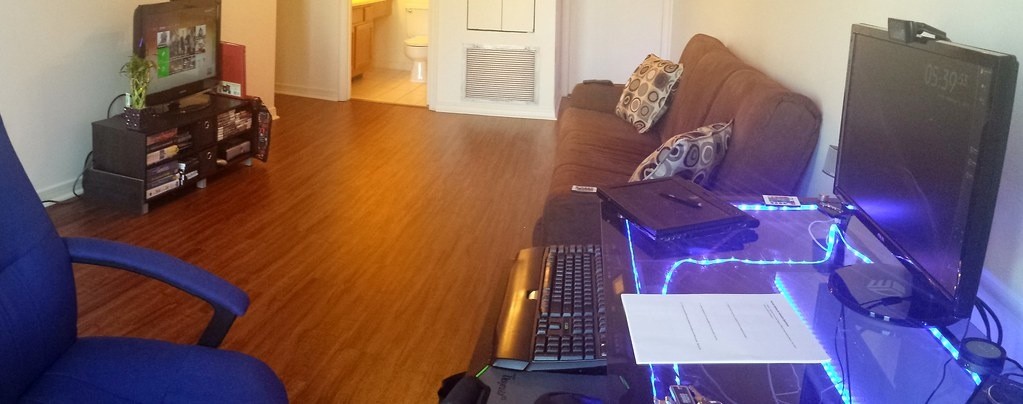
[659,192,702,207]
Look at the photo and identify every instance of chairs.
[0,116,291,404]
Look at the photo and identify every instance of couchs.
[532,30,822,250]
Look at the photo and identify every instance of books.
[146,125,199,200]
[217,109,252,142]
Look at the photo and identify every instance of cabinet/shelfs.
[84,94,256,215]
[352,2,392,75]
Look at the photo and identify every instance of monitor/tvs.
[133,0,222,114]
[828,22,1019,327]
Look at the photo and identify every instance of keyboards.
[489,242,607,372]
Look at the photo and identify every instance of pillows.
[628,117,734,182]
[614,52,686,134]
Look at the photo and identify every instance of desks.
[597,192,1023,404]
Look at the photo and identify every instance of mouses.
[535,392,604,404]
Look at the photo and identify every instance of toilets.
[403,7,427,83]
